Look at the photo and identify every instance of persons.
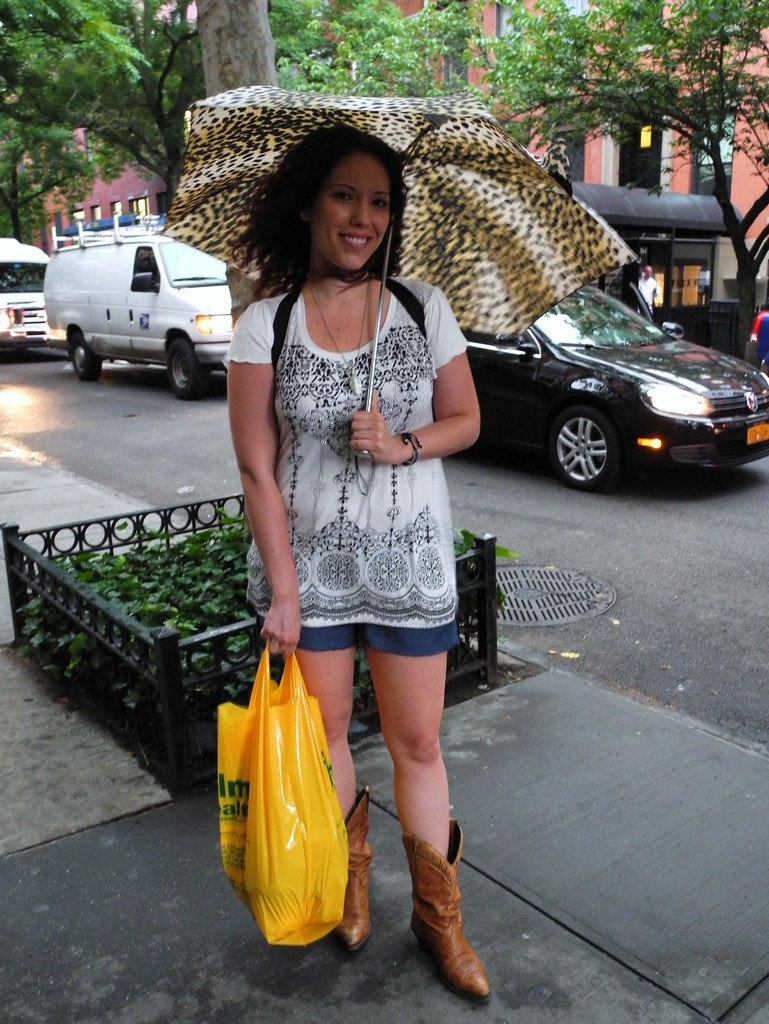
[223,122,496,1000]
[698,260,712,304]
[638,265,661,316]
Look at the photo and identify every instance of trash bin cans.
[708,299,740,357]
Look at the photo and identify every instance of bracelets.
[399,432,425,466]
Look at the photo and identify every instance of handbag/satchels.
[218,636,349,947]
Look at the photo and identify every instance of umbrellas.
[160,83,640,460]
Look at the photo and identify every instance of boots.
[335,785,372,950]
[402,819,491,998]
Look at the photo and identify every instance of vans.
[0,237,49,358]
[43,209,234,403]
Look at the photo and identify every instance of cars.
[454,281,769,495]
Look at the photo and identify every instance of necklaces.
[307,277,370,395]
